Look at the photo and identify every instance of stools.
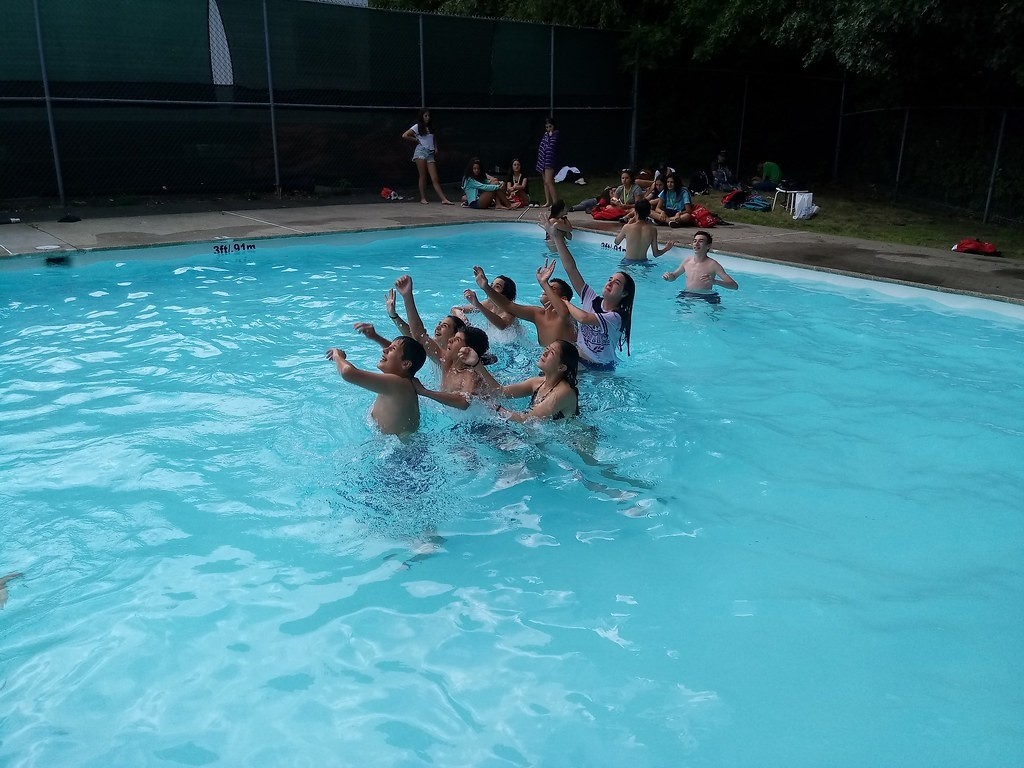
[772,187,809,215]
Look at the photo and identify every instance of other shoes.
[618,216,628,224]
[574,180,587,186]
[532,201,540,208]
[669,221,680,228]
[529,200,535,208]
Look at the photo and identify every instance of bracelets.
[389,314,398,319]
[497,405,501,411]
[472,358,480,367]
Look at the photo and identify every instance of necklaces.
[623,186,626,203]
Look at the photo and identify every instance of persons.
[452,275,517,328]
[535,118,561,207]
[663,231,738,293]
[460,158,520,210]
[401,108,455,205]
[385,290,463,354]
[751,160,781,190]
[458,339,580,420]
[571,164,694,229]
[545,199,573,241]
[712,152,731,180]
[473,258,577,345]
[536,212,636,365]
[394,274,489,410]
[325,322,422,434]
[506,158,531,205]
[614,198,673,260]
